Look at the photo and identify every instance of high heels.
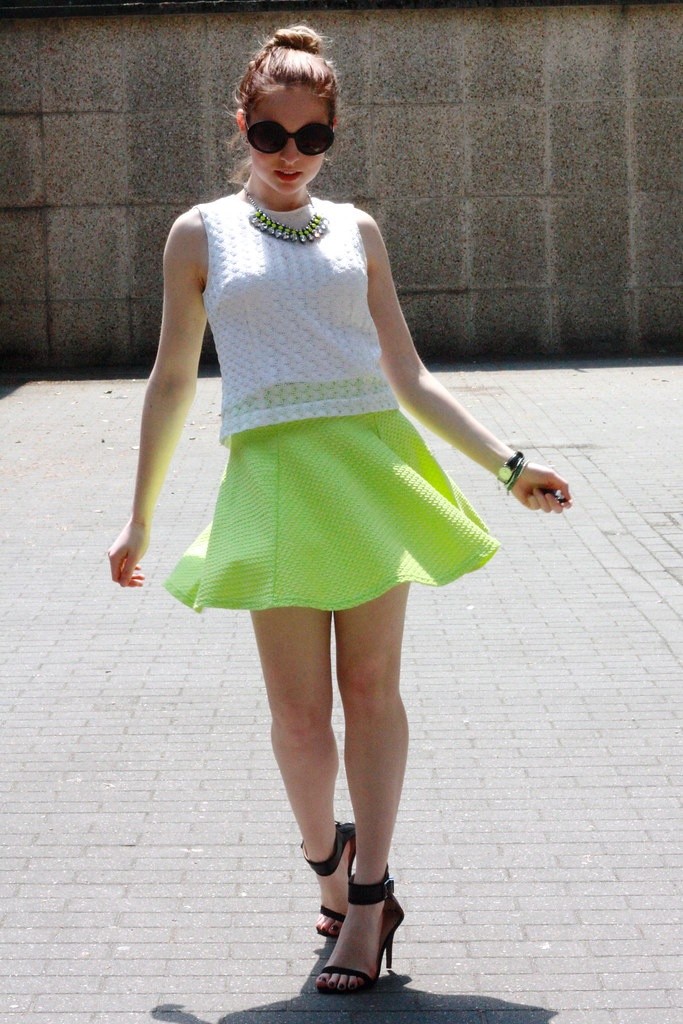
[316,864,404,994]
[300,820,356,938]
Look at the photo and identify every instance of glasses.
[244,112,336,156]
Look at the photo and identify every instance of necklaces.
[243,182,330,243]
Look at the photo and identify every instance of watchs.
[497,450,524,484]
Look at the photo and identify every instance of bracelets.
[505,458,531,491]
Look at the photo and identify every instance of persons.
[106,21,579,994]
